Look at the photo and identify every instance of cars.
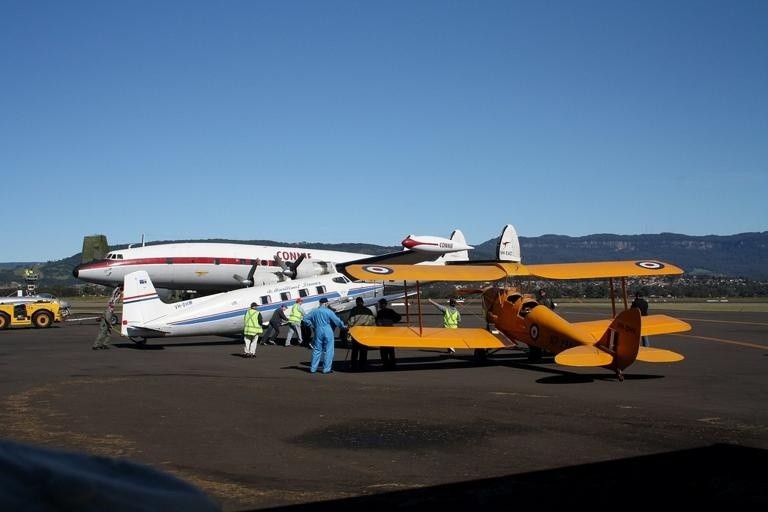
[0,289,72,318]
[0,299,61,330]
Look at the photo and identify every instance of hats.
[319,297,329,303]
[295,298,303,302]
[356,297,363,302]
[281,304,286,309]
[377,298,386,303]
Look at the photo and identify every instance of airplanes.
[73,226,522,293]
[119,224,474,339]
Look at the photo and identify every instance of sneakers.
[259,341,267,346]
[269,339,275,344]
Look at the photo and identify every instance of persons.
[284,298,305,347]
[91,302,115,350]
[259,303,288,346]
[630,292,649,347]
[426,298,462,354]
[302,298,347,374]
[307,302,338,351]
[241,302,263,358]
[345,297,375,372]
[535,289,555,312]
[375,298,401,370]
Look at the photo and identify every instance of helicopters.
[333,259,692,382]
